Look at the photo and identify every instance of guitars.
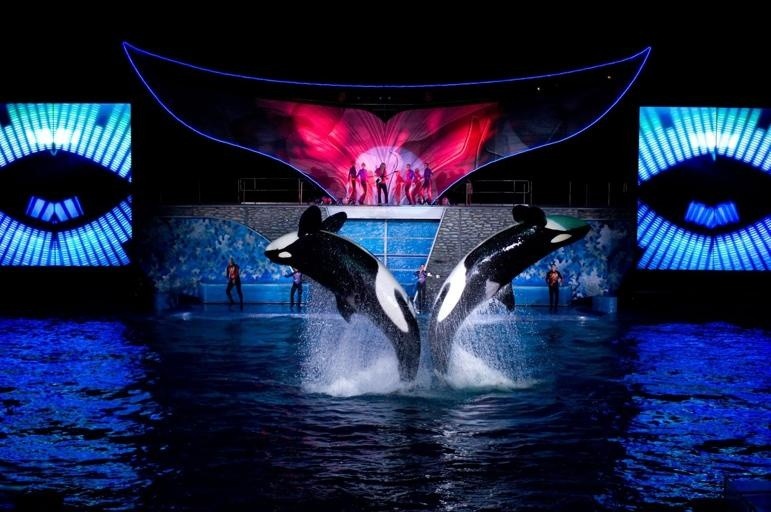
[376,171,398,183]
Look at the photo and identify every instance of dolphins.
[426,202,594,391]
[262,202,422,394]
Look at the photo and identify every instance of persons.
[316,162,448,206]
[225,256,243,306]
[413,263,428,314]
[465,178,474,207]
[283,268,304,308]
[545,264,563,314]
